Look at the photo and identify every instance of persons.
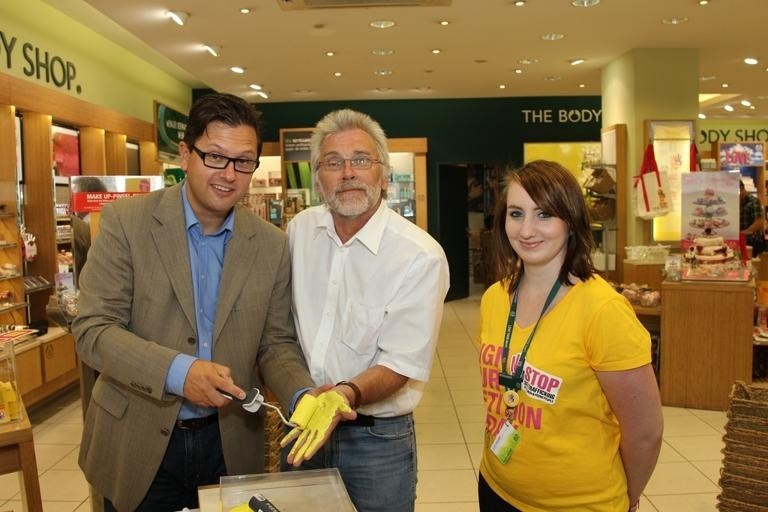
[71,176,109,220]
[69,91,337,511]
[278,107,453,512]
[476,159,666,511]
[739,180,766,259]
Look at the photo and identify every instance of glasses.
[190,143,261,175]
[317,156,387,171]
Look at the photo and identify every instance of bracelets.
[336,380,361,409]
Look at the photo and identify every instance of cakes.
[683,236,734,264]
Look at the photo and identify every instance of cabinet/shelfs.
[0,75,160,417]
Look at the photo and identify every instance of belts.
[174,414,217,431]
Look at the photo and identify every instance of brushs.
[217,387,319,430]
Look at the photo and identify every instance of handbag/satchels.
[636,170,674,220]
